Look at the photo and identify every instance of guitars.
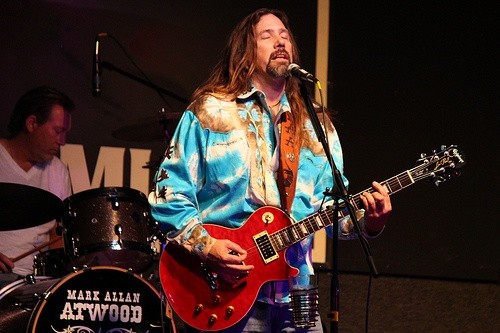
[158,142,466,331]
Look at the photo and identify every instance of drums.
[0,267,177,333]
[59,187,156,275]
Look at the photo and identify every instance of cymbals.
[0,182,63,231]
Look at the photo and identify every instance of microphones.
[93,38,102,97]
[287,64,319,84]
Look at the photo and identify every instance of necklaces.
[269,101,280,108]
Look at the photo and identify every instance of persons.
[0,86,75,284]
[148,8,392,333]
